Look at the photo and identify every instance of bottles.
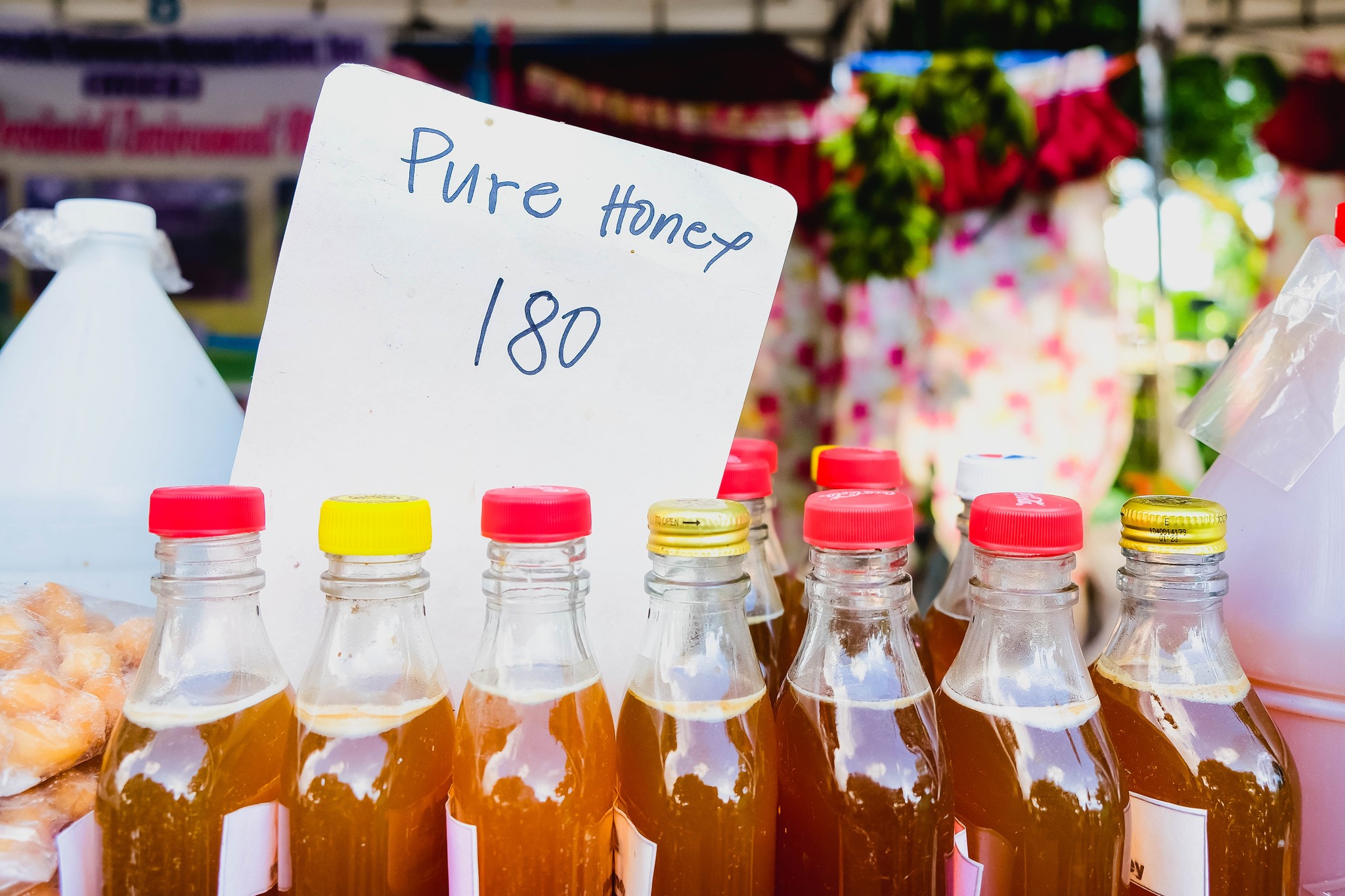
[444,485,616,896]
[773,489,955,896]
[1087,495,1303,896]
[716,438,1050,709]
[612,499,777,896]
[92,484,297,896]
[278,496,458,896]
[1191,201,1345,896]
[0,199,246,607]
[934,492,1132,896]
